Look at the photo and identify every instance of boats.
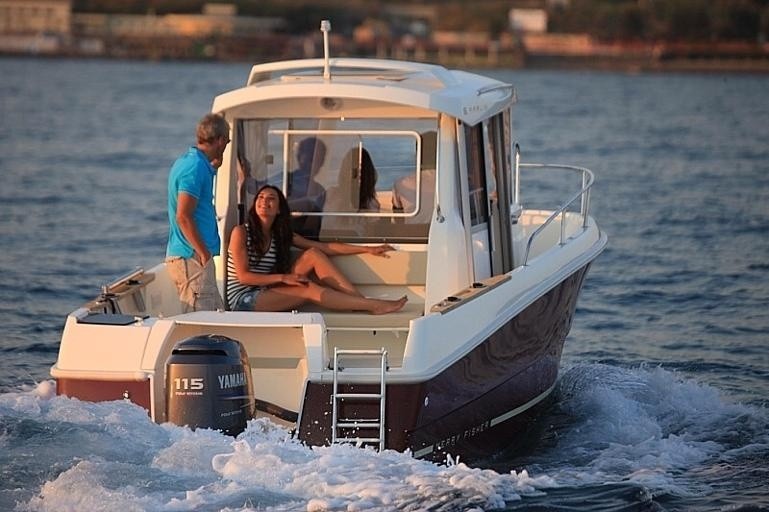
[51,13,608,461]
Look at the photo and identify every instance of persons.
[165,110,231,316]
[241,137,327,242]
[225,183,409,315]
[316,146,391,242]
[391,130,440,225]
